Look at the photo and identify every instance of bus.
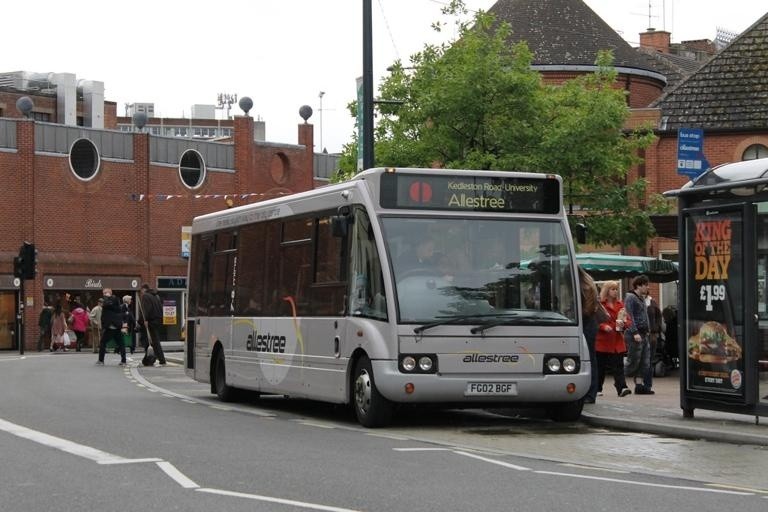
[183,165,596,431]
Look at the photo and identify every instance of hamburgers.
[689,322,741,363]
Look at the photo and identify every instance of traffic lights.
[13,242,38,280]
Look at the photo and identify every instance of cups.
[615,320,624,331]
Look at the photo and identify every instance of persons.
[592,273,677,399]
[95,288,127,366]
[136,283,167,368]
[395,236,453,282]
[562,262,601,404]
[35,282,146,355]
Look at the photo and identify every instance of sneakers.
[596,384,654,397]
